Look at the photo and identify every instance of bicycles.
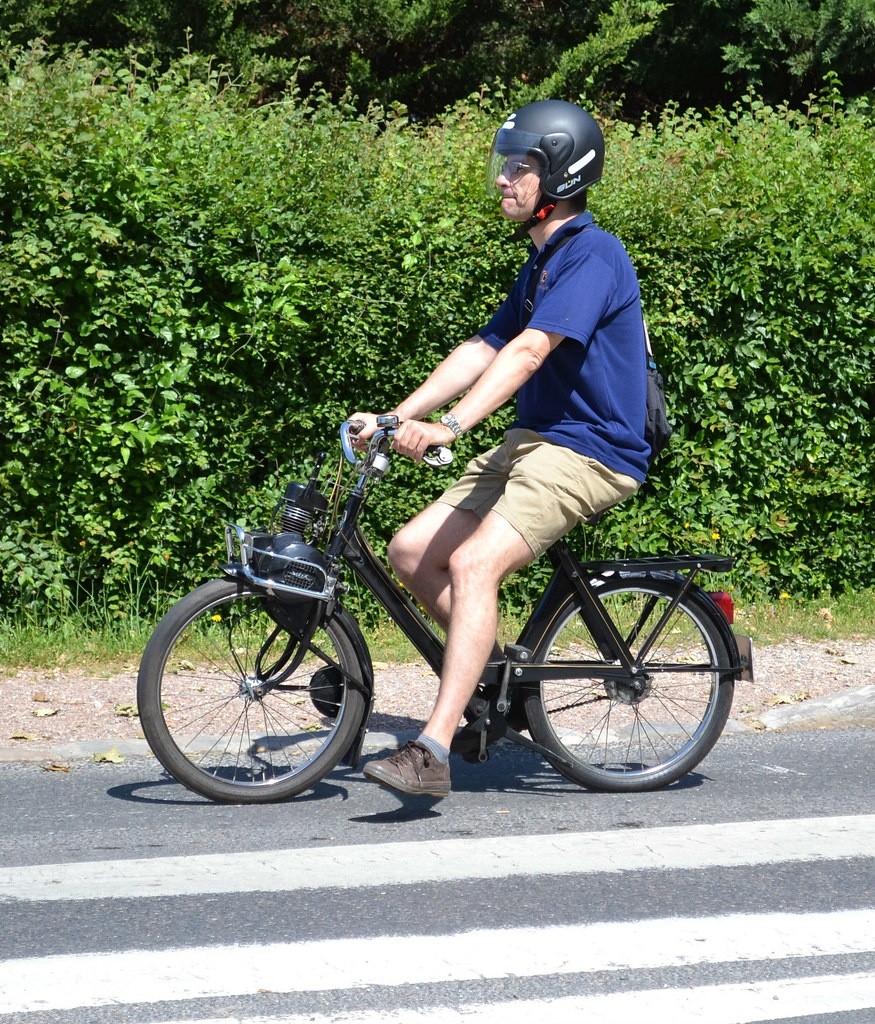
[136,415,755,804]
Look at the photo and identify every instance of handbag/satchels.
[519,225,673,460]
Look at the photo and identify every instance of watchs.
[440,412,463,439]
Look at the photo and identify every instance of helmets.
[491,99,606,201]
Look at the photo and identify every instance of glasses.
[500,160,542,177]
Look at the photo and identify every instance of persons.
[345,100,653,799]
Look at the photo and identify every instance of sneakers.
[363,739,451,798]
